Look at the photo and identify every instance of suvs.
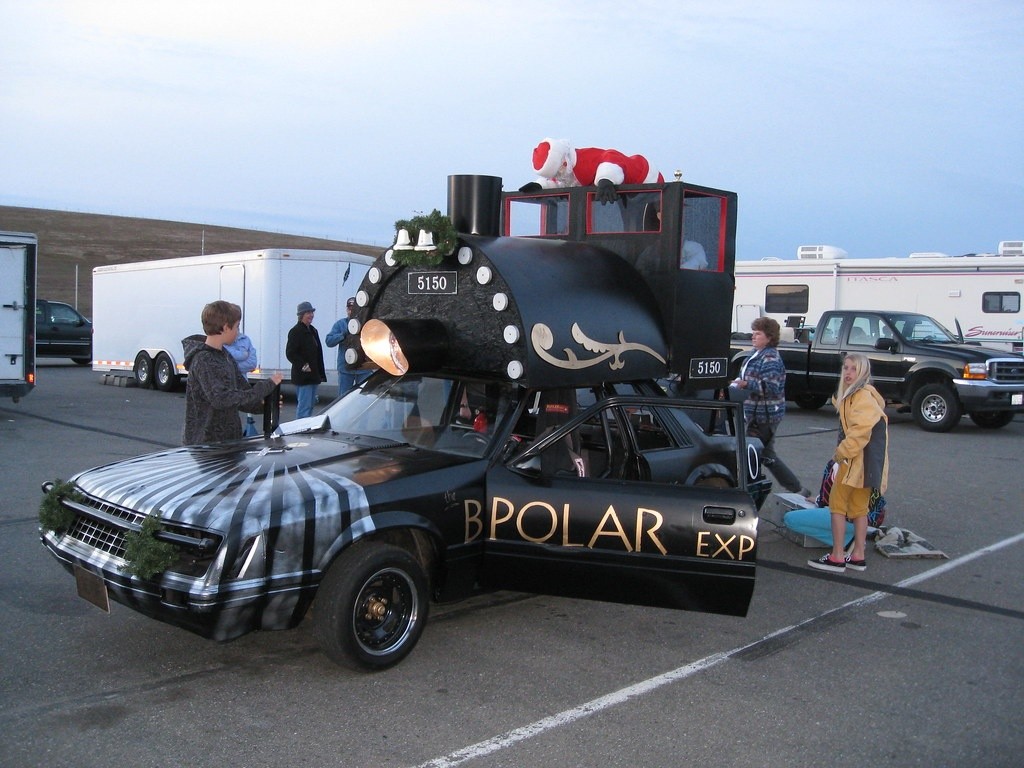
[36,297,94,367]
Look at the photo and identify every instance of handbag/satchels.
[746,420,774,447]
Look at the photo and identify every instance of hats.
[296,302,316,315]
[346,298,355,305]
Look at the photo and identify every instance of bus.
[731,240,1024,360]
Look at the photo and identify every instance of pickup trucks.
[729,310,1024,433]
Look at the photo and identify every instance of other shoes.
[796,486,811,498]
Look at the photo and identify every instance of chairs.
[535,387,580,476]
[848,327,871,344]
[883,325,893,339]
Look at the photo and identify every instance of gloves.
[301,363,311,372]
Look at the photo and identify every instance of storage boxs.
[770,493,830,547]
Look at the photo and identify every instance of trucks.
[0,231,38,403]
[92,248,379,393]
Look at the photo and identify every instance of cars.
[35,174,775,674]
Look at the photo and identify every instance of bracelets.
[460,403,469,408]
[302,363,309,372]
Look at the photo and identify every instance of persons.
[730,317,812,499]
[414,376,470,426]
[635,211,709,270]
[325,297,373,398]
[222,323,257,382]
[182,299,284,446]
[285,301,328,420]
[518,139,665,206]
[807,354,889,573]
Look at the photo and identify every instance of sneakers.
[845,556,867,571]
[808,553,846,572]
